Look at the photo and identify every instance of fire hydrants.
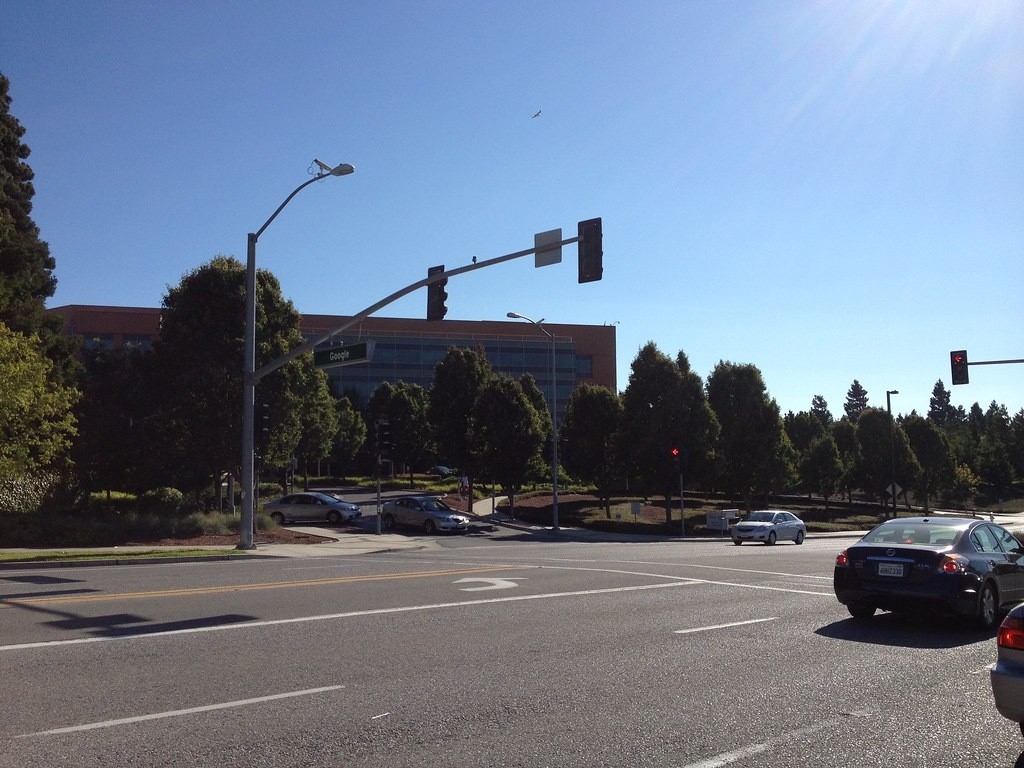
[989,512,995,523]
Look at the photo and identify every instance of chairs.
[913,527,930,542]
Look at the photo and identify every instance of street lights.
[237,162,359,553]
[508,312,564,529]
[884,389,900,518]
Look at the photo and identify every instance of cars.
[830,519,1024,629]
[381,496,469,535]
[733,509,807,547]
[430,465,454,479]
[260,491,364,525]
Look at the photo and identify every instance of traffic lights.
[672,448,686,477]
[950,350,971,385]
[427,265,451,324]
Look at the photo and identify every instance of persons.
[462,475,469,500]
[989,514,995,521]
